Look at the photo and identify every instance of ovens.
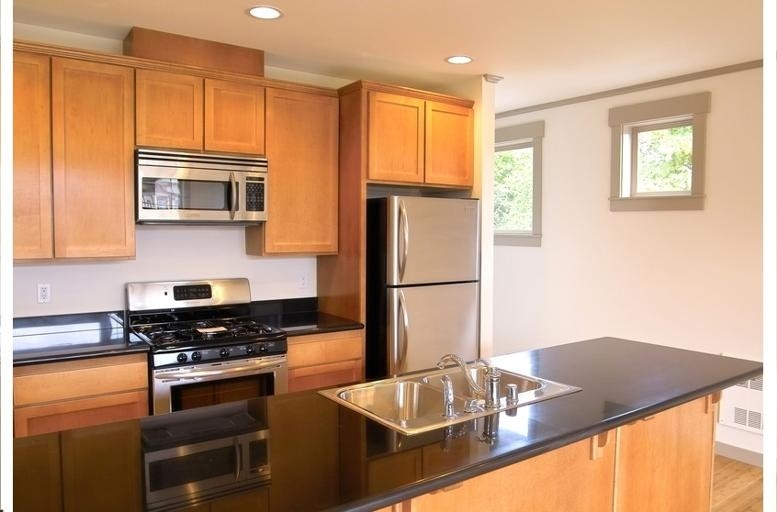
[150,338,288,415]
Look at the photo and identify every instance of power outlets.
[37,284,49,302]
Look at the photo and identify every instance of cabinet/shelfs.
[266,80,339,254]
[286,330,363,391]
[13,418,142,512]
[136,68,264,155]
[364,435,471,497]
[367,90,475,190]
[182,485,271,512]
[13,49,135,261]
[267,391,339,512]
[14,353,148,438]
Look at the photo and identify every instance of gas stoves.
[125,315,287,365]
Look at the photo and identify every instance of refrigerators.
[367,196,482,377]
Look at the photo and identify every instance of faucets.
[437,354,501,409]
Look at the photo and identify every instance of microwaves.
[137,150,270,223]
[139,411,273,511]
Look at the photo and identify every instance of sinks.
[317,379,488,436]
[398,362,583,411]
